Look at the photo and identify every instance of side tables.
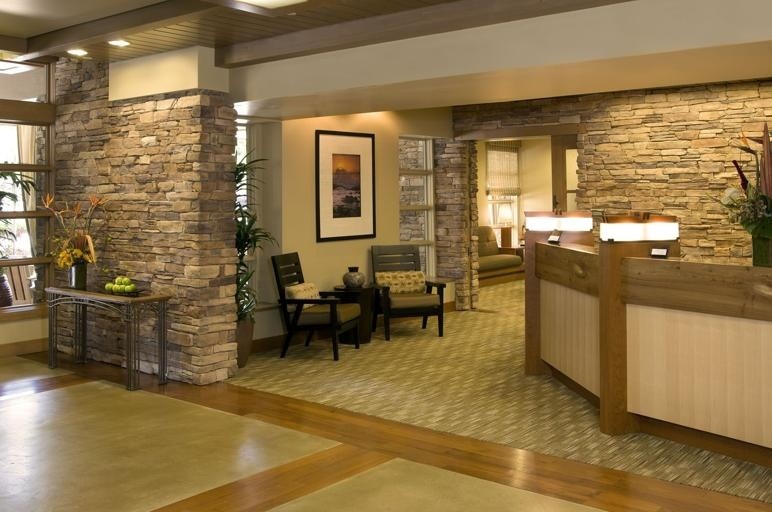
[334,285,380,345]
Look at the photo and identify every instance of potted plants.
[234,148,279,368]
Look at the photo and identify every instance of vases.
[68,264,87,289]
[752,216,772,268]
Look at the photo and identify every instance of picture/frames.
[315,130,376,243]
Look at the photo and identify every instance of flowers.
[37,189,107,267]
[705,123,772,237]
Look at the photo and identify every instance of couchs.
[478,226,525,286]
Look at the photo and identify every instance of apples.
[105,276,136,292]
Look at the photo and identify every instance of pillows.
[285,282,320,312]
[0,274,14,307]
[375,271,425,294]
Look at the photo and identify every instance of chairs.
[271,252,362,361]
[0,256,55,358]
[371,244,447,341]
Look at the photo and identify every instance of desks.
[44,285,172,391]
